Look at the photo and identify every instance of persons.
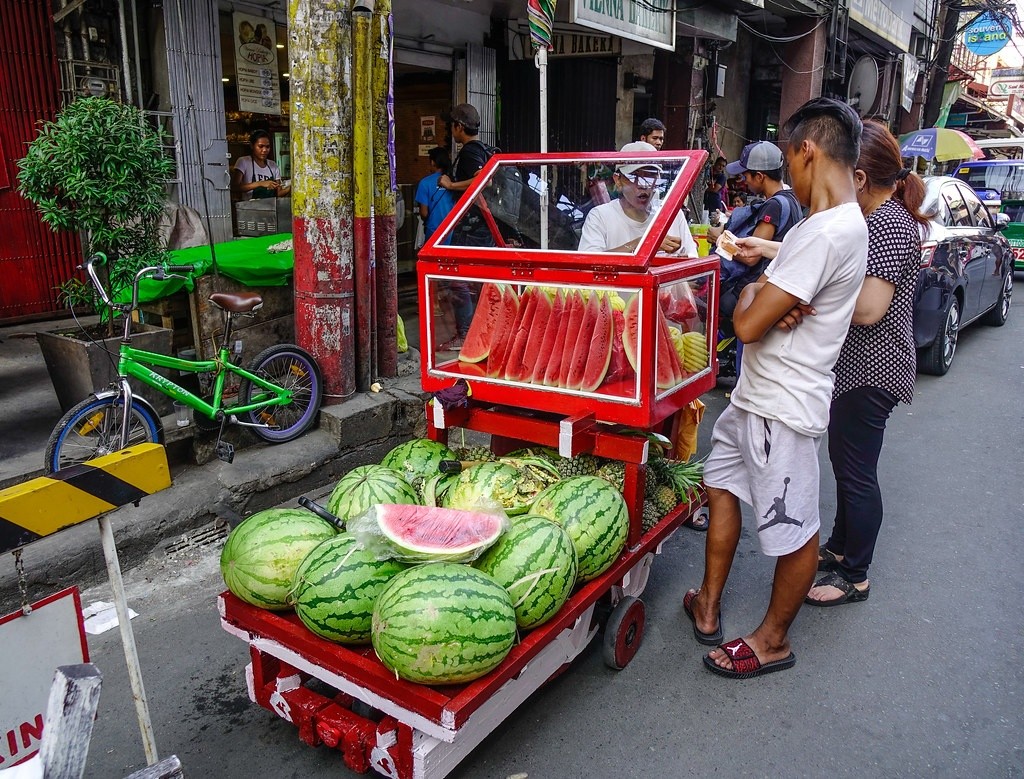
[577,117,710,531]
[804,119,935,607]
[684,97,869,677]
[415,104,497,351]
[703,141,805,386]
[231,130,291,201]
[992,237,1011,275]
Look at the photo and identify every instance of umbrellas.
[899,128,986,175]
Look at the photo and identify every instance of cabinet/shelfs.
[226,118,267,145]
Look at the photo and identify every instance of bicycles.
[42,250,324,478]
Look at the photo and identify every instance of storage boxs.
[415,149,721,427]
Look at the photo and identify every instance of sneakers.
[437,333,465,350]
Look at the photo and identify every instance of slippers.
[815,544,844,571]
[803,571,870,606]
[704,637,797,679]
[682,588,725,646]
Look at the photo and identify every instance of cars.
[891,173,1015,378]
[946,137,1024,271]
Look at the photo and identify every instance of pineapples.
[524,285,708,373]
[455,445,712,534]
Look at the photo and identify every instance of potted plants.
[13,96,174,426]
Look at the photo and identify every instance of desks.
[99,233,295,348]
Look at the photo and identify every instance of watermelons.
[458,282,686,389]
[220,438,629,684]
[459,362,485,377]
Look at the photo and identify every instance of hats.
[428,147,449,166]
[441,104,481,130]
[726,140,785,174]
[616,141,665,176]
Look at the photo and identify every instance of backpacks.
[445,141,523,226]
[708,194,791,279]
